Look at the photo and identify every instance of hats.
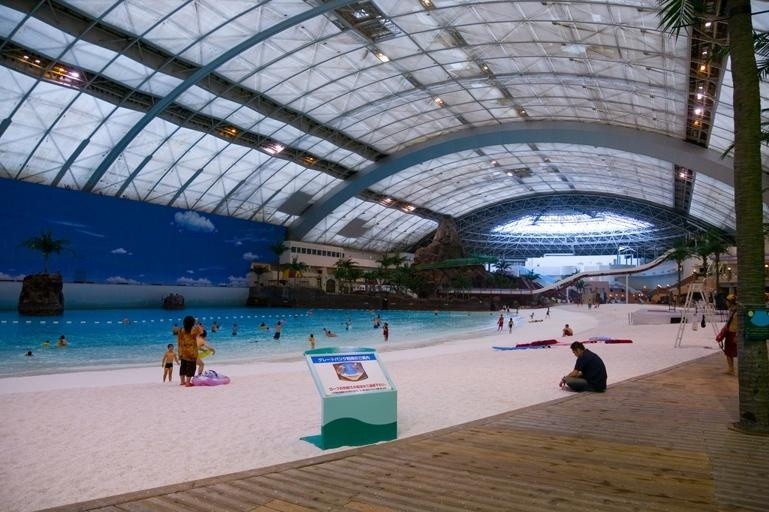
[727,295,736,302]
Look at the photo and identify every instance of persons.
[25,335,68,356]
[716,294,739,377]
[176,316,216,388]
[162,344,180,382]
[323,328,338,337]
[558,341,608,393]
[232,323,240,336]
[258,321,283,339]
[172,321,219,336]
[381,296,390,310]
[432,294,678,337]
[309,334,315,348]
[372,315,389,342]
[346,320,352,330]
